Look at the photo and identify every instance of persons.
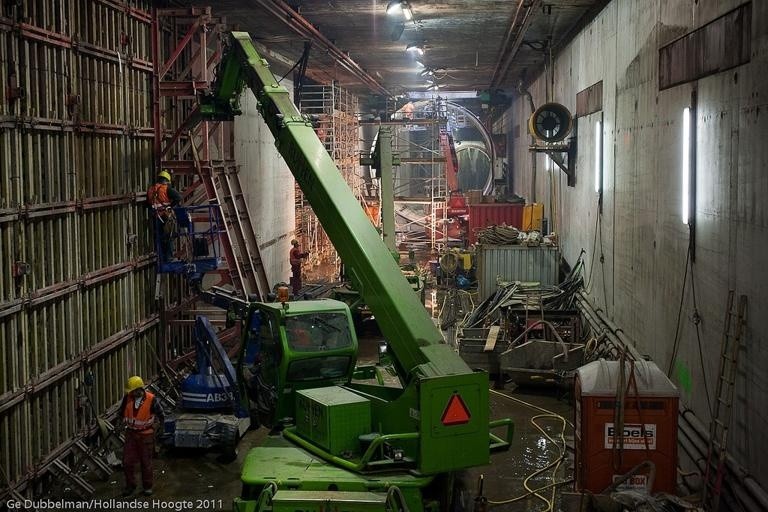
[285,315,316,359]
[146,168,183,260]
[117,376,164,496]
[290,239,309,294]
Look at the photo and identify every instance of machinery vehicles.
[186,30,520,512]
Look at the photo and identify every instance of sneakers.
[144,488,154,496]
[122,485,134,497]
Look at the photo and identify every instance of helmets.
[124,376,144,393]
[291,239,298,245]
[158,171,171,181]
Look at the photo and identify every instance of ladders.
[702,291,746,512]
